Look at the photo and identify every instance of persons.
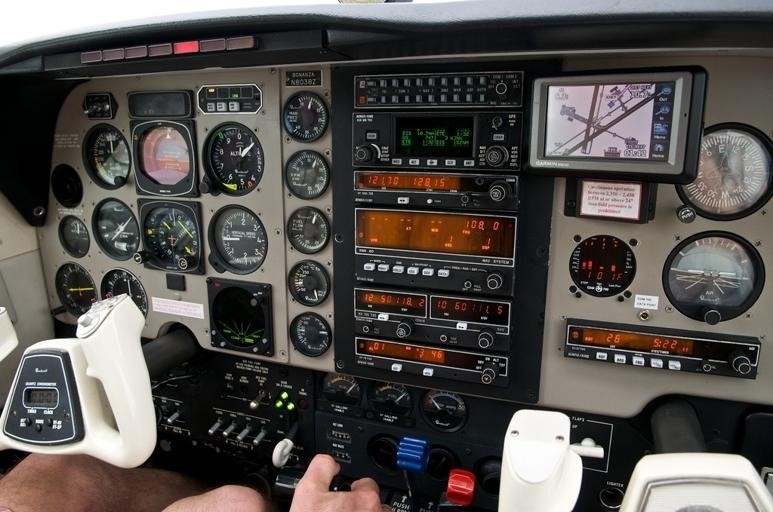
[0,453,393,512]
